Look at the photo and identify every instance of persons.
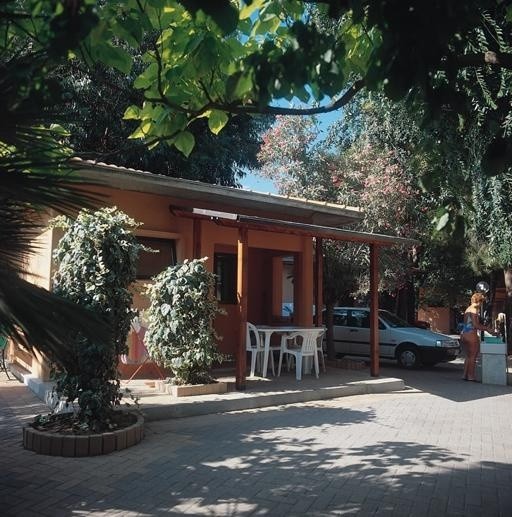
[461,291,498,380]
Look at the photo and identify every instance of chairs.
[242,321,328,381]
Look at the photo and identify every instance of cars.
[312,306,462,367]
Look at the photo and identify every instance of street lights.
[475,280,491,342]
